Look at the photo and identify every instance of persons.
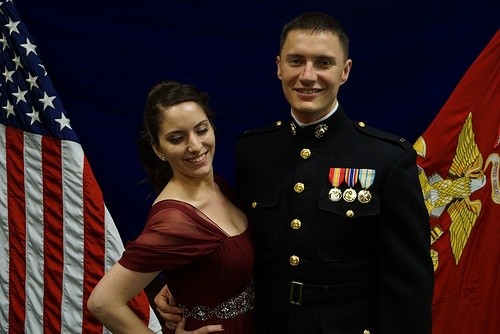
[151,11,434,334]
[85,81,260,333]
